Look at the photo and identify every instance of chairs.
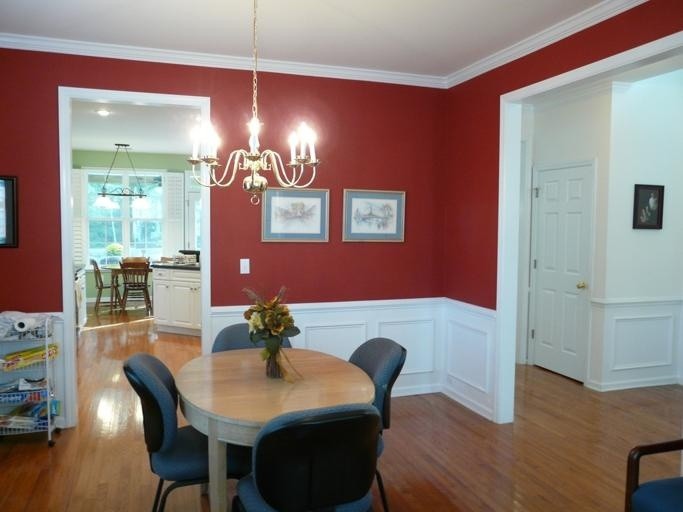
[117,261,151,318]
[232,402,389,512]
[349,336,406,458]
[122,353,253,512]
[210,322,292,352]
[624,437,683,512]
[121,257,150,263]
[90,259,122,312]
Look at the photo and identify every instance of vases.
[265,348,284,378]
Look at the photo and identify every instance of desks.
[100,264,153,314]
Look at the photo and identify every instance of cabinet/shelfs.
[170,270,202,331]
[152,268,171,326]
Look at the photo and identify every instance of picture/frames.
[633,184,665,230]
[0,175,19,248]
[342,189,406,243]
[260,187,330,243]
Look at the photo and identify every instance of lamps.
[96,109,110,117]
[90,143,150,211]
[186,0,321,205]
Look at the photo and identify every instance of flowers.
[242,285,301,361]
